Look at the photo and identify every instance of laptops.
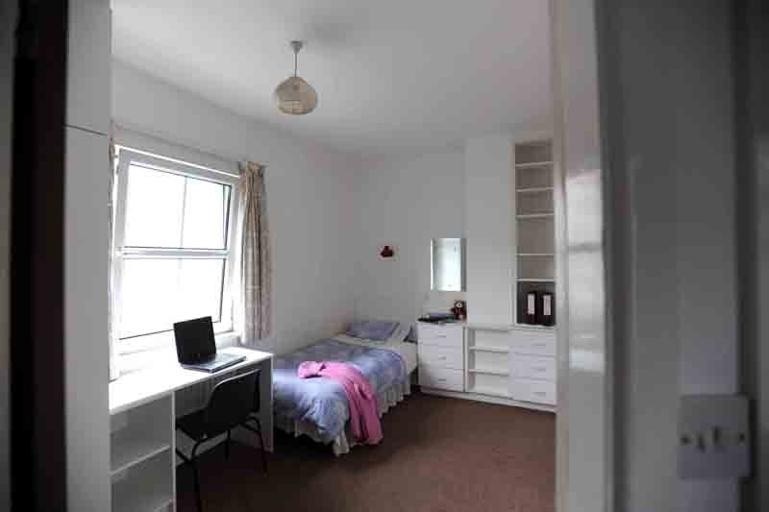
[173,316,246,372]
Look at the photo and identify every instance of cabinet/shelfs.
[416,135,556,416]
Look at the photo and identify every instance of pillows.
[346,318,412,343]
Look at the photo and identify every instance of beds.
[271,316,417,457]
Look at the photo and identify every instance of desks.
[109,346,274,512]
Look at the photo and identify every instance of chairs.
[176,368,265,512]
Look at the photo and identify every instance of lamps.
[269,40,319,116]
